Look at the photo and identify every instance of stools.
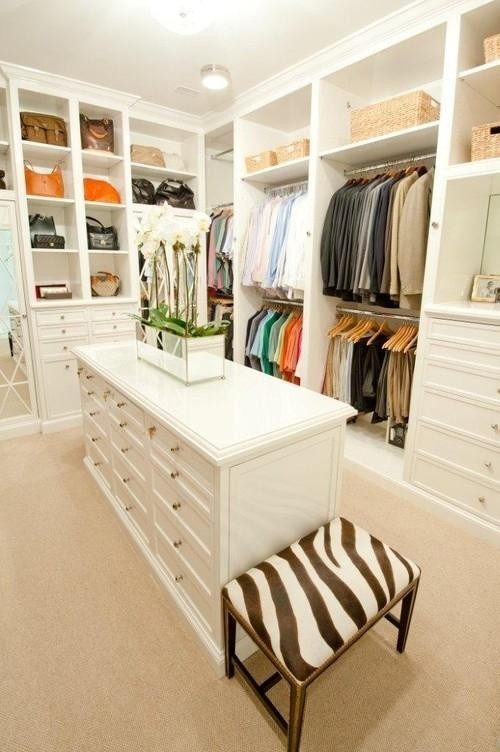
[219,514,422,751]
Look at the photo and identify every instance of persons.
[478,281,495,297]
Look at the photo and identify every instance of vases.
[130,318,227,387]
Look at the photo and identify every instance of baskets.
[471,122,500,161]
[351,90,440,143]
[484,34,500,63]
[245,138,310,173]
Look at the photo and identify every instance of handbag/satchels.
[29,213,65,248]
[131,144,165,168]
[21,112,67,146]
[132,178,194,209]
[24,159,64,198]
[91,272,120,296]
[80,113,114,152]
[86,216,118,250]
[83,178,121,204]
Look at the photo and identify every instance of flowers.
[130,204,231,335]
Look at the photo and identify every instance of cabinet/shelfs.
[0,84,204,302]
[66,335,358,688]
[204,22,499,530]
[34,306,137,424]
